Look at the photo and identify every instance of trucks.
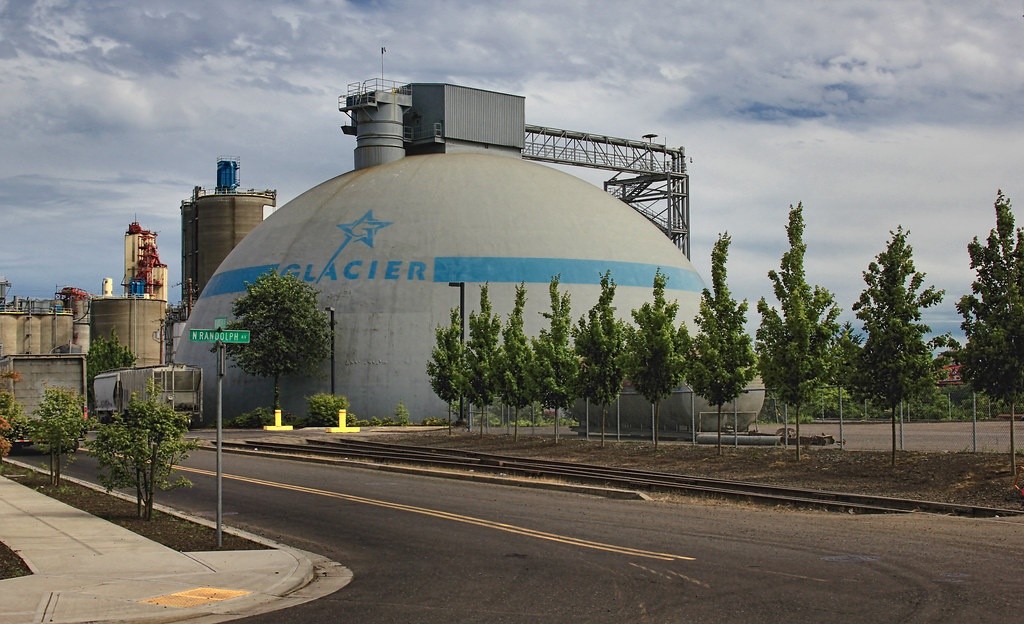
[92,363,204,431]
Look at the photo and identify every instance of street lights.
[327,306,336,397]
[450,281,466,424]
